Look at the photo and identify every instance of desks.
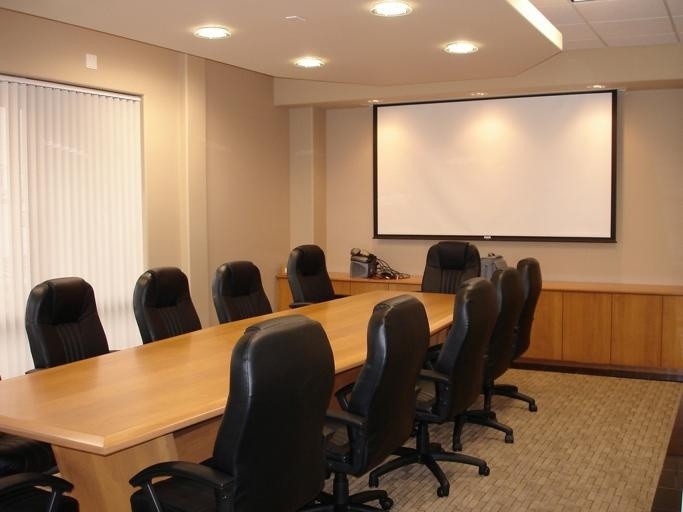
[0,290,458,512]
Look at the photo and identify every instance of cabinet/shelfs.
[275,273,423,314]
[514,281,682,382]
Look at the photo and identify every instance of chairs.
[435,265,526,452]
[369,277,499,497]
[286,245,351,309]
[466,257,543,423]
[24,277,122,375]
[128,313,336,511]
[132,267,203,344]
[300,294,430,510]
[0,376,61,488]
[414,242,482,295]
[211,261,274,324]
[0,472,80,511]
